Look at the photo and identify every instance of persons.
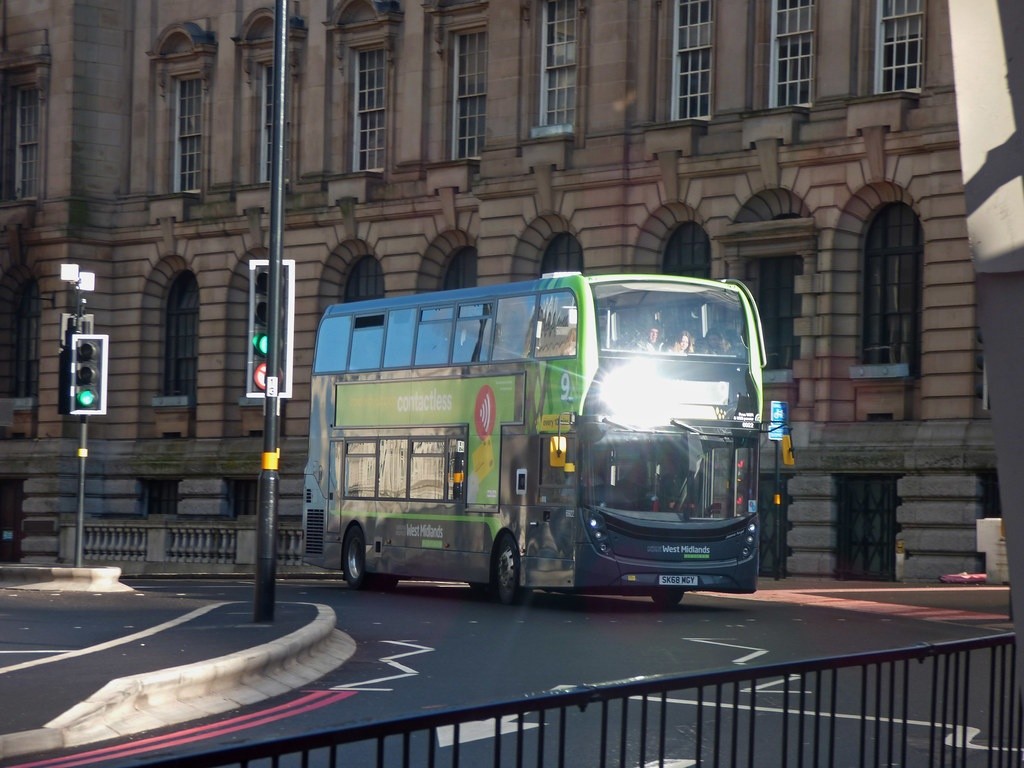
[627,322,731,355]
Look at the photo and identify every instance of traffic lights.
[69,334,112,416]
[246,259,292,401]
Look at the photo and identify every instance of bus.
[298,274,798,607]
